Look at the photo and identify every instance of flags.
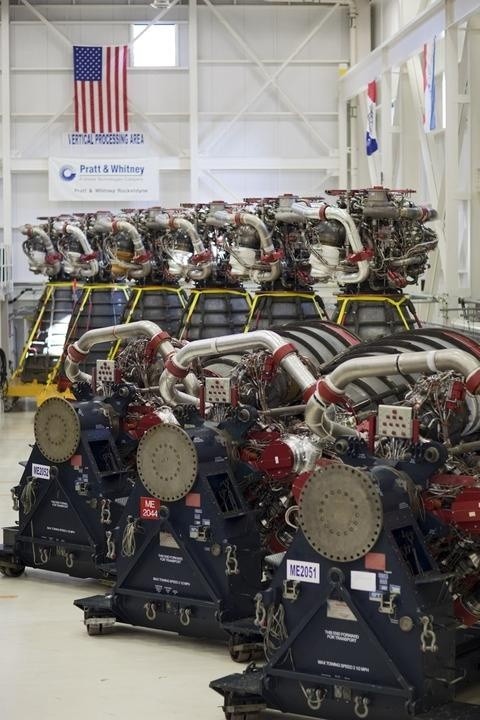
[72,44,129,135]
[367,81,378,156]
